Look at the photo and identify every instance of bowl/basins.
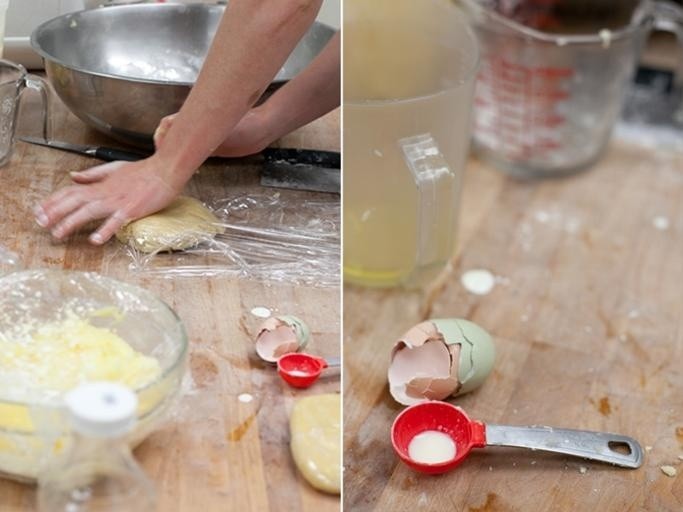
[30,0,340,137]
[0,267,188,487]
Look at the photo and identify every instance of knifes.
[21,129,147,170]
[260,148,341,196]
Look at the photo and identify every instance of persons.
[31,0,340,247]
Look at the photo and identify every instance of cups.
[0,58,54,169]
[340,0,681,290]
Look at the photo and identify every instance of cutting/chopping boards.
[344,132,683,512]
[0,52,342,512]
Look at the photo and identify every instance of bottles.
[37,385,157,511]
